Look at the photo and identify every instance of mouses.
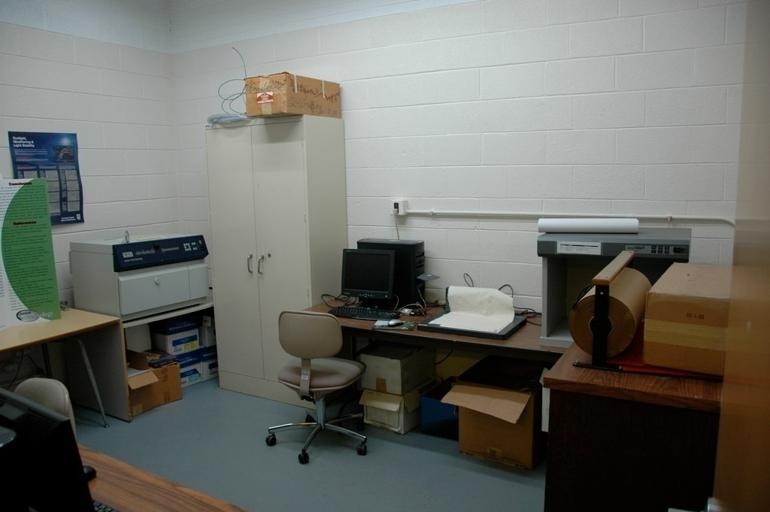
[388,317,405,326]
[82,465,95,478]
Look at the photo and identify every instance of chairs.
[264,311,371,464]
[14,377,77,440]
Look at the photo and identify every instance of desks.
[541,341,722,512]
[0,308,133,424]
[77,444,249,512]
[303,298,568,356]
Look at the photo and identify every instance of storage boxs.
[360,382,438,434]
[442,384,541,470]
[130,306,217,417]
[244,72,341,119]
[360,342,437,394]
[641,261,732,376]
[421,356,509,442]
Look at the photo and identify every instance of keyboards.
[93,498,116,512]
[327,307,400,321]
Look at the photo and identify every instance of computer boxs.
[358,237,425,307]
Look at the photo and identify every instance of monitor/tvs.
[340,248,393,308]
[1,386,94,512]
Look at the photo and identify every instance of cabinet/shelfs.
[206,114,347,411]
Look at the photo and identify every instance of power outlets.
[391,200,407,216]
